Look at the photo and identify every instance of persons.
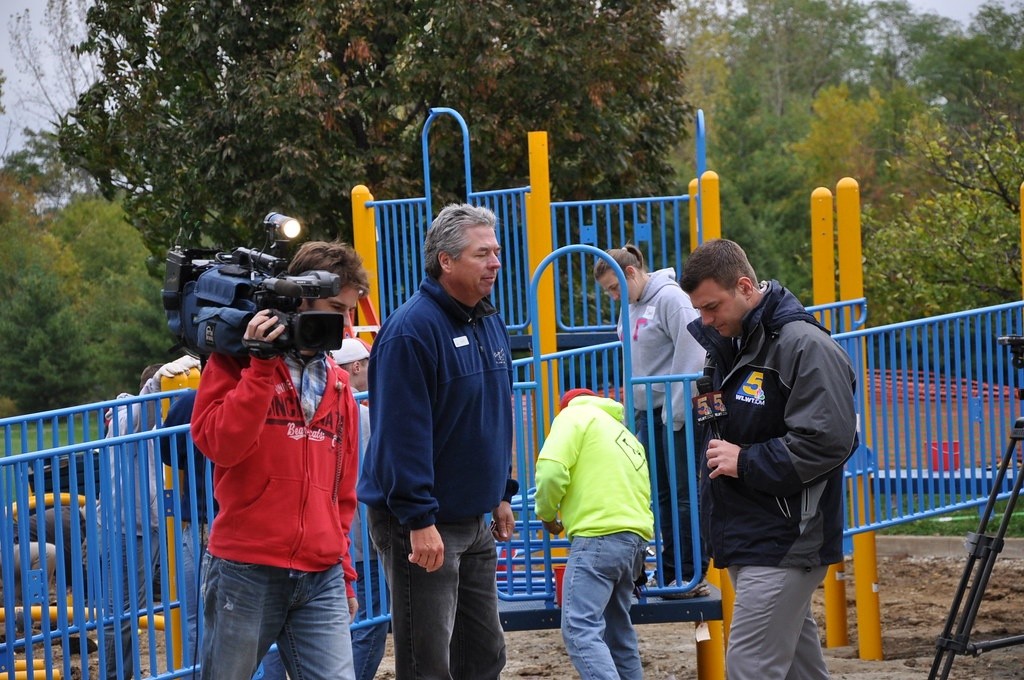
[0,500,97,606]
[679,239,858,680]
[193,241,370,680]
[158,388,219,668]
[356,203,520,680]
[594,244,710,600]
[98,355,201,680]
[329,338,389,680]
[534,389,654,680]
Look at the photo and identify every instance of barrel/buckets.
[497,549,517,581]
[926,441,960,471]
[553,566,565,607]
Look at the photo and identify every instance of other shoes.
[663,576,711,598]
[646,579,660,589]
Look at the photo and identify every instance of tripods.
[927,334,1024,680]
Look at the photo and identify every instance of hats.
[559,384,597,413]
[331,337,372,365]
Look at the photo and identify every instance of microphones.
[263,278,303,299]
[692,375,729,443]
